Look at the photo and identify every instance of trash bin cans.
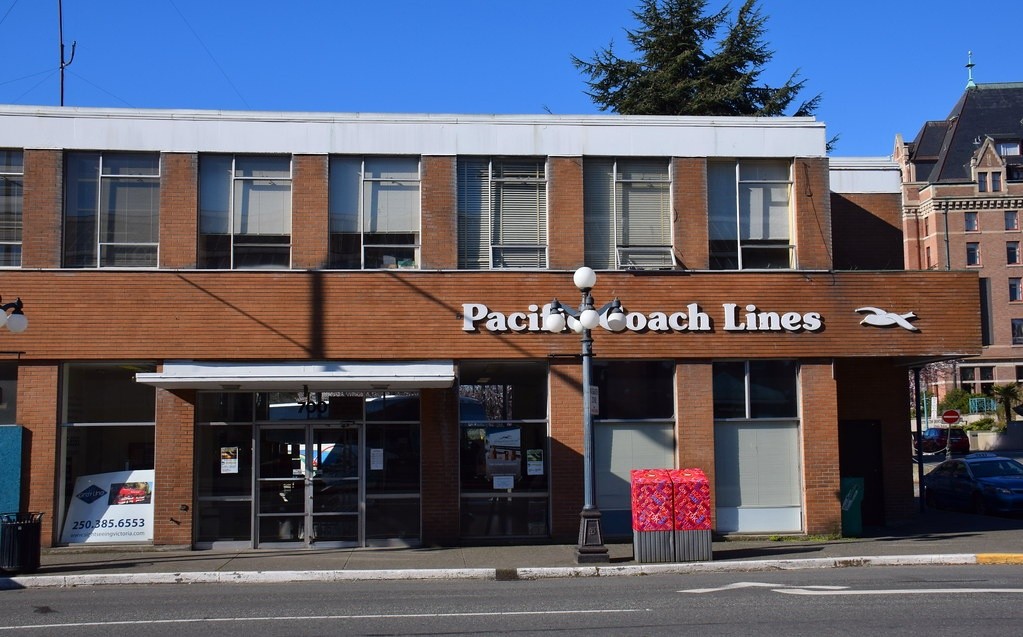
[0,512,45,573]
[667,467,712,562]
[629,469,675,563]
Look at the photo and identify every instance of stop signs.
[942,409,960,425]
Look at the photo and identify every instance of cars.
[923,452,1023,515]
[915,427,970,455]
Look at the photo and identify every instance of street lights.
[542,266,627,568]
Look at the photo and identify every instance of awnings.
[134,371,456,389]
